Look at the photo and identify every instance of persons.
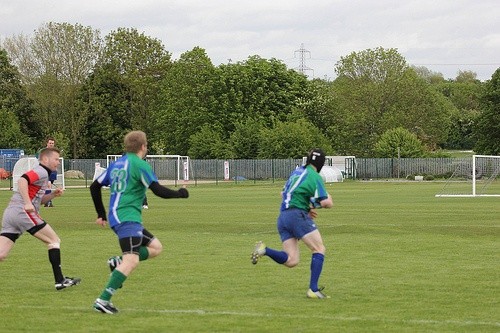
[0,147,82,292]
[88,131,190,315]
[35,138,56,207]
[249,148,335,300]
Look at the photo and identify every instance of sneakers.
[250,239,266,265]
[54,276,83,290]
[108,255,122,288]
[143,204,148,209]
[306,285,331,299]
[93,298,120,315]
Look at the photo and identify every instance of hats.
[302,149,325,173]
